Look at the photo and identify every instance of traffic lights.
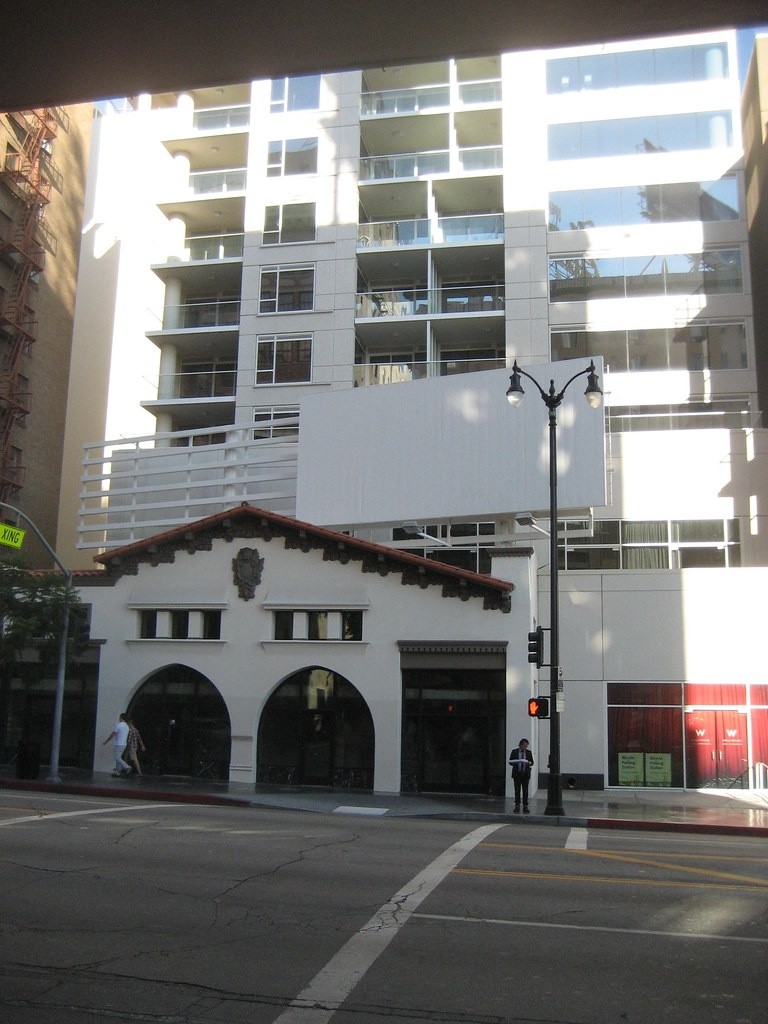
[529,698,548,717]
[528,626,544,669]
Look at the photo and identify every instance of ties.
[521,752,523,771]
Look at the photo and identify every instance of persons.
[113,720,146,777]
[509,738,534,812]
[103,713,132,777]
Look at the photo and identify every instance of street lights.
[506,358,603,816]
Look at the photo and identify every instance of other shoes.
[135,774,143,777]
[513,807,520,813]
[126,766,132,774]
[523,807,530,813]
[111,773,120,776]
[113,768,124,772]
